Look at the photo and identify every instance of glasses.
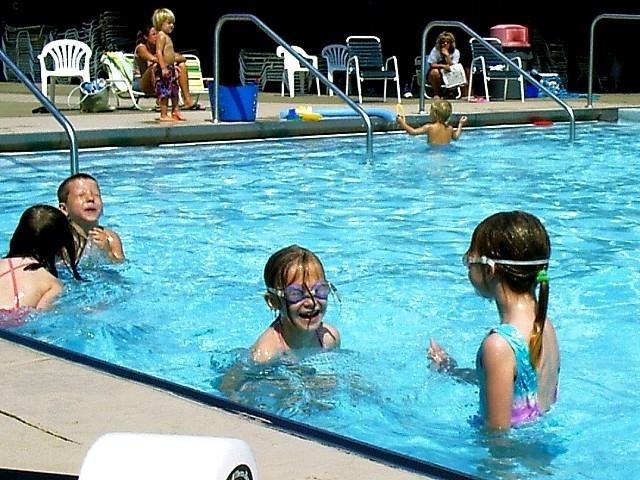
[463,252,549,267]
[268,280,330,303]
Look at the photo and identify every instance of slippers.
[181,103,206,110]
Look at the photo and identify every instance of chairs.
[529,38,569,89]
[239,35,403,105]
[468,37,525,103]
[410,56,462,100]
[0,0,121,112]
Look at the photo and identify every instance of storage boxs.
[210,82,259,121]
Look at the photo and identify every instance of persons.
[0,207,80,332]
[423,32,468,99]
[131,8,206,126]
[426,211,560,430]
[250,246,340,364]
[58,174,127,264]
[397,98,469,146]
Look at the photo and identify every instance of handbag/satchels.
[80,81,109,111]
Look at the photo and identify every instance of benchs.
[99,48,216,112]
